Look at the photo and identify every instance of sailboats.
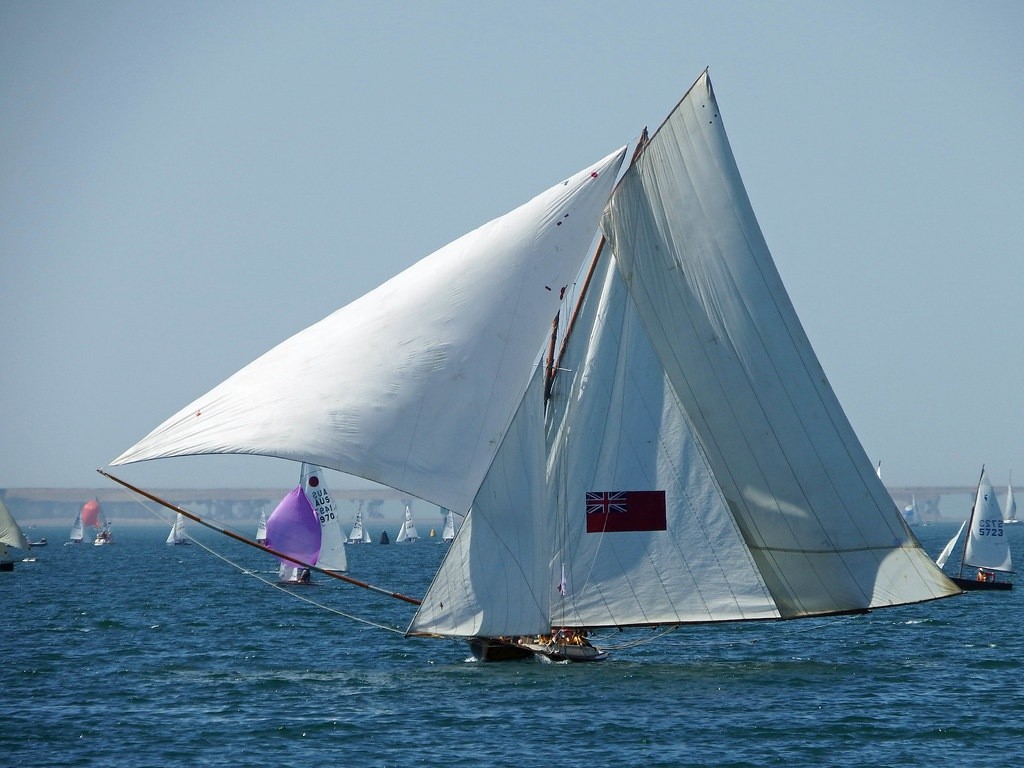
[394,503,423,545]
[0,497,33,573]
[999,481,1022,528]
[904,493,927,527]
[165,506,192,548]
[346,502,373,546]
[252,504,272,548]
[269,460,350,585]
[927,461,1016,595]
[63,505,91,548]
[434,507,460,548]
[97,64,969,671]
[82,500,114,547]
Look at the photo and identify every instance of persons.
[976,566,996,582]
[499,628,596,649]
